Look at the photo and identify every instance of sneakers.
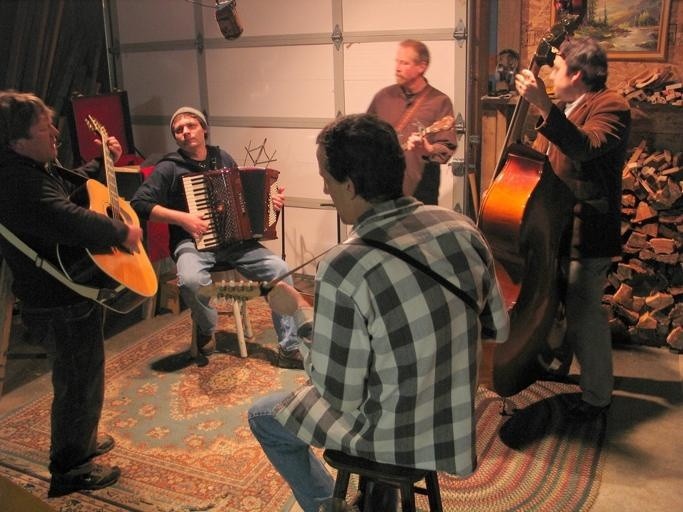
[191,336,217,356]
[277,348,305,370]
[48,431,120,498]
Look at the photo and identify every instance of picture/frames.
[550,1,670,62]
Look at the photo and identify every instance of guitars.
[400,115,456,151]
[56,113,158,314]
[198,280,315,307]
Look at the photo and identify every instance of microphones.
[213,1,243,41]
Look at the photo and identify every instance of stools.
[323,450,445,512]
[189,264,253,360]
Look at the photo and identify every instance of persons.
[514,36,635,426]
[126,105,311,369]
[361,38,459,205]
[247,112,511,512]
[0,90,144,496]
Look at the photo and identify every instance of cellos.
[477,1,587,397]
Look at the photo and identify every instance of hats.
[170,106,207,131]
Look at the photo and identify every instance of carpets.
[1,288,606,509]
